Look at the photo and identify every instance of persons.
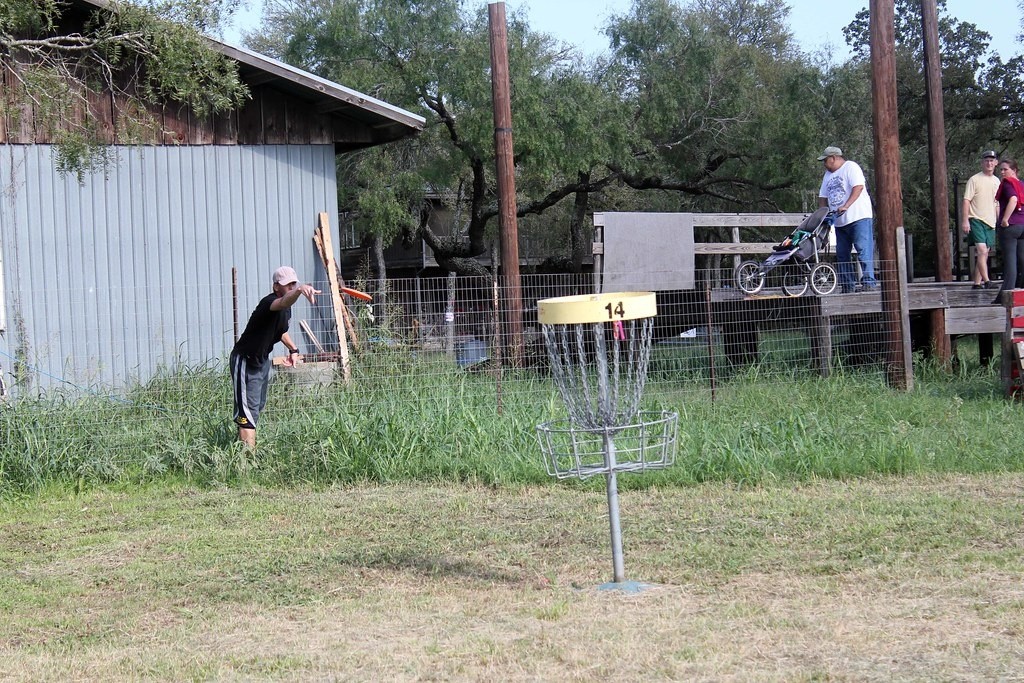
[229,265,322,457]
[816,147,878,293]
[989,158,1024,305]
[962,149,1001,290]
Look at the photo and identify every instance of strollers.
[733,206,839,298]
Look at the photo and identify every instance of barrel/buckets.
[455,342,488,367]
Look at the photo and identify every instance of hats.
[983,149,996,158]
[272,266,299,286]
[817,146,843,160]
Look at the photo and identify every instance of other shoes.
[972,284,983,289]
[991,299,1002,304]
[840,288,856,293]
[861,284,876,291]
[985,281,999,289]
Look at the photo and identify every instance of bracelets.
[288,348,300,354]
